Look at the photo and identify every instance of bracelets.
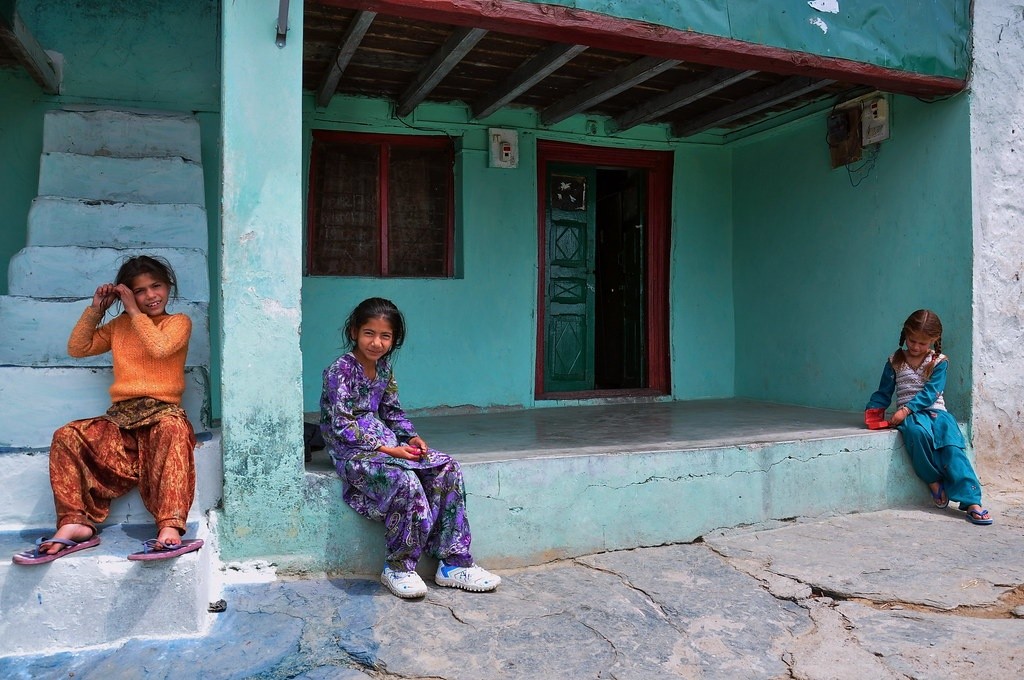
[904,407,909,414]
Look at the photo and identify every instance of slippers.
[11,534,100,565]
[965,509,994,525]
[127,538,204,561]
[930,482,950,509]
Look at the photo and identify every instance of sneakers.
[380,563,428,599]
[435,559,502,592]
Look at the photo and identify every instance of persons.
[320,298,501,597]
[865,310,993,524]
[13,255,204,565]
